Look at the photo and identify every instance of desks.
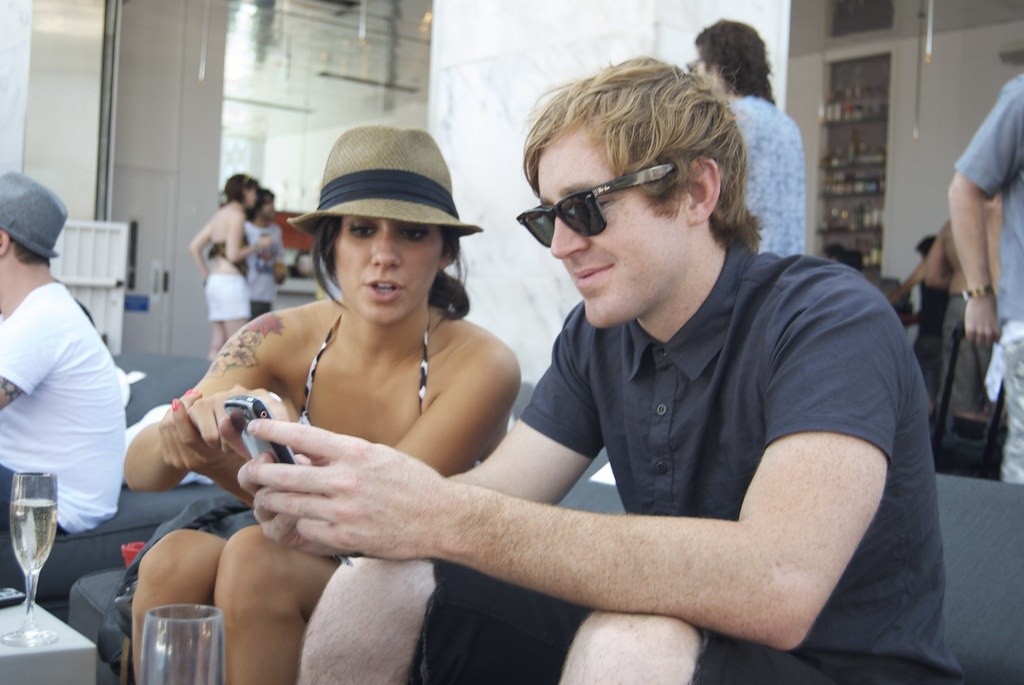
[0,600,97,685]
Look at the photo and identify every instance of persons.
[123,124,524,685]
[884,70,1024,486]
[0,170,132,540]
[216,56,967,685]
[684,18,809,265]
[187,172,291,365]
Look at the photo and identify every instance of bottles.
[814,78,885,271]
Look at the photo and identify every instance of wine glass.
[1,472,60,646]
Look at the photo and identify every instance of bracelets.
[961,284,994,302]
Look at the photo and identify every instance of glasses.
[517,161,679,249]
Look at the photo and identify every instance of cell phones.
[224,396,295,466]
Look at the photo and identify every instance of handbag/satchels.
[109,490,277,640]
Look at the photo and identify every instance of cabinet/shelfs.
[821,114,888,273]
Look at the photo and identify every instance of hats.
[286,123,483,239]
[0,173,68,258]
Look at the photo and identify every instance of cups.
[137,602,227,685]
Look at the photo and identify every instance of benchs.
[0,351,227,625]
[74,482,1024,685]
